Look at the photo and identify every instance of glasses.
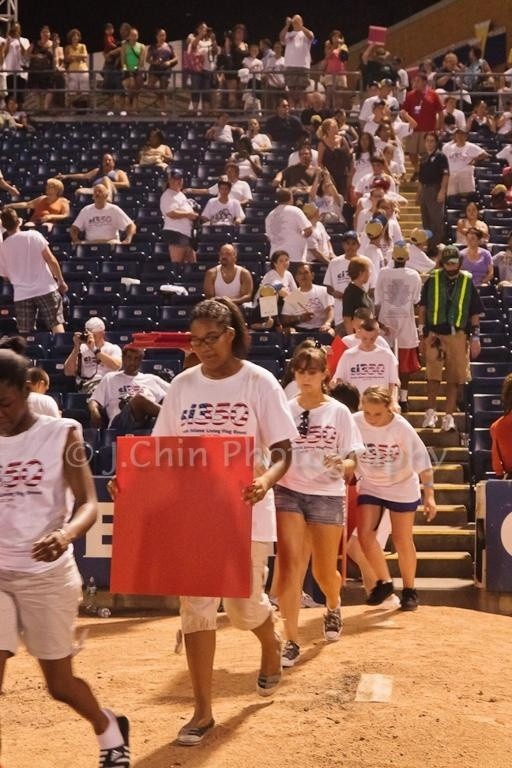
[299,410,309,436]
[189,327,227,348]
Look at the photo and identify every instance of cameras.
[224,31,235,37]
[290,19,296,24]
[78,332,89,343]
[207,28,213,31]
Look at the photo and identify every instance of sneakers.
[256,631,284,697]
[177,716,214,747]
[281,640,300,668]
[323,598,343,642]
[422,408,438,428]
[401,588,418,611]
[366,579,394,606]
[398,398,408,414]
[97,716,130,768]
[441,414,457,432]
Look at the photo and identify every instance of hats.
[369,78,402,115]
[372,177,390,192]
[454,126,467,134]
[410,228,434,245]
[339,230,360,243]
[168,169,186,180]
[303,202,317,217]
[441,245,460,264]
[393,239,409,263]
[366,214,387,239]
[85,317,106,333]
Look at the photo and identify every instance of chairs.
[435,125,512,517]
[0,119,349,479]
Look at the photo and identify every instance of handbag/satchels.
[168,52,178,68]
[479,71,496,90]
[334,147,350,170]
[418,163,432,184]
